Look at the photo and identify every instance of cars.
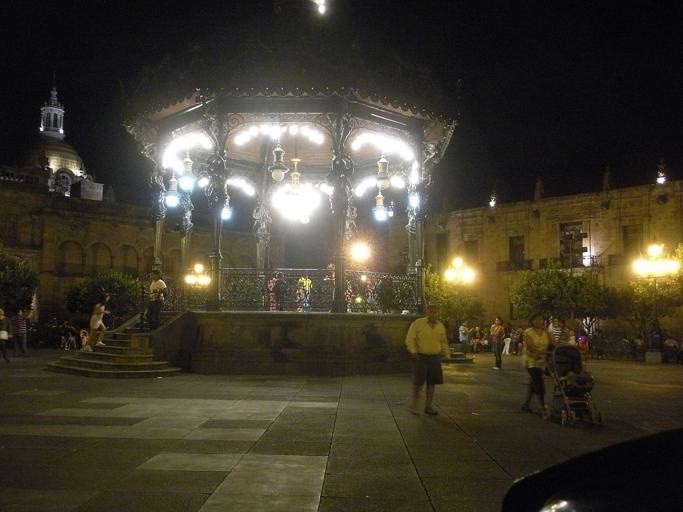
[498,427,683,512]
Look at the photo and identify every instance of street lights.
[183,262,211,297]
[629,243,679,364]
[441,257,477,352]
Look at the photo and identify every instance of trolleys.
[543,345,606,429]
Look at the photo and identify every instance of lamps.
[177,150,196,191]
[372,188,386,221]
[376,153,391,190]
[163,171,181,207]
[268,138,289,181]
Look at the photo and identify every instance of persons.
[455,316,523,370]
[267,271,313,312]
[522,312,553,416]
[150,270,167,323]
[345,264,418,314]
[323,263,336,312]
[86,293,111,352]
[547,311,683,393]
[0,307,86,357]
[406,305,451,416]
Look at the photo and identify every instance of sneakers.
[96,341,106,346]
[84,345,93,352]
[424,406,438,415]
[492,364,500,371]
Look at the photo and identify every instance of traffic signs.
[561,234,571,239]
[560,249,569,254]
[572,247,587,254]
[571,232,588,239]
